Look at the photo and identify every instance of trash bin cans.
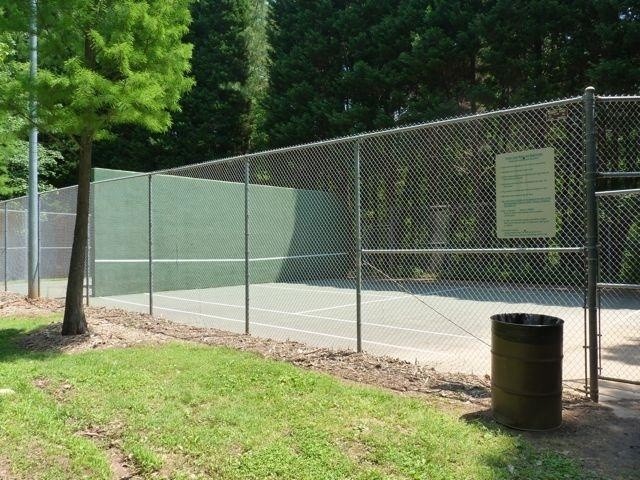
[493,315,563,431]
